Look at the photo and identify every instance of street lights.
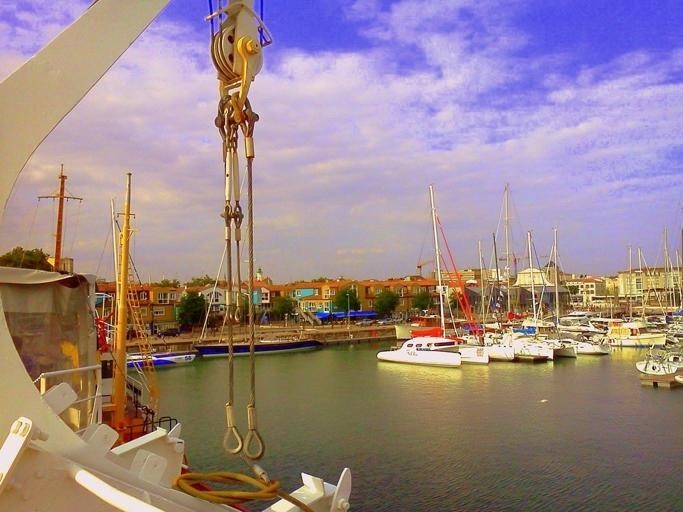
[345,294,352,329]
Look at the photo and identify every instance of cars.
[356,317,375,327]
